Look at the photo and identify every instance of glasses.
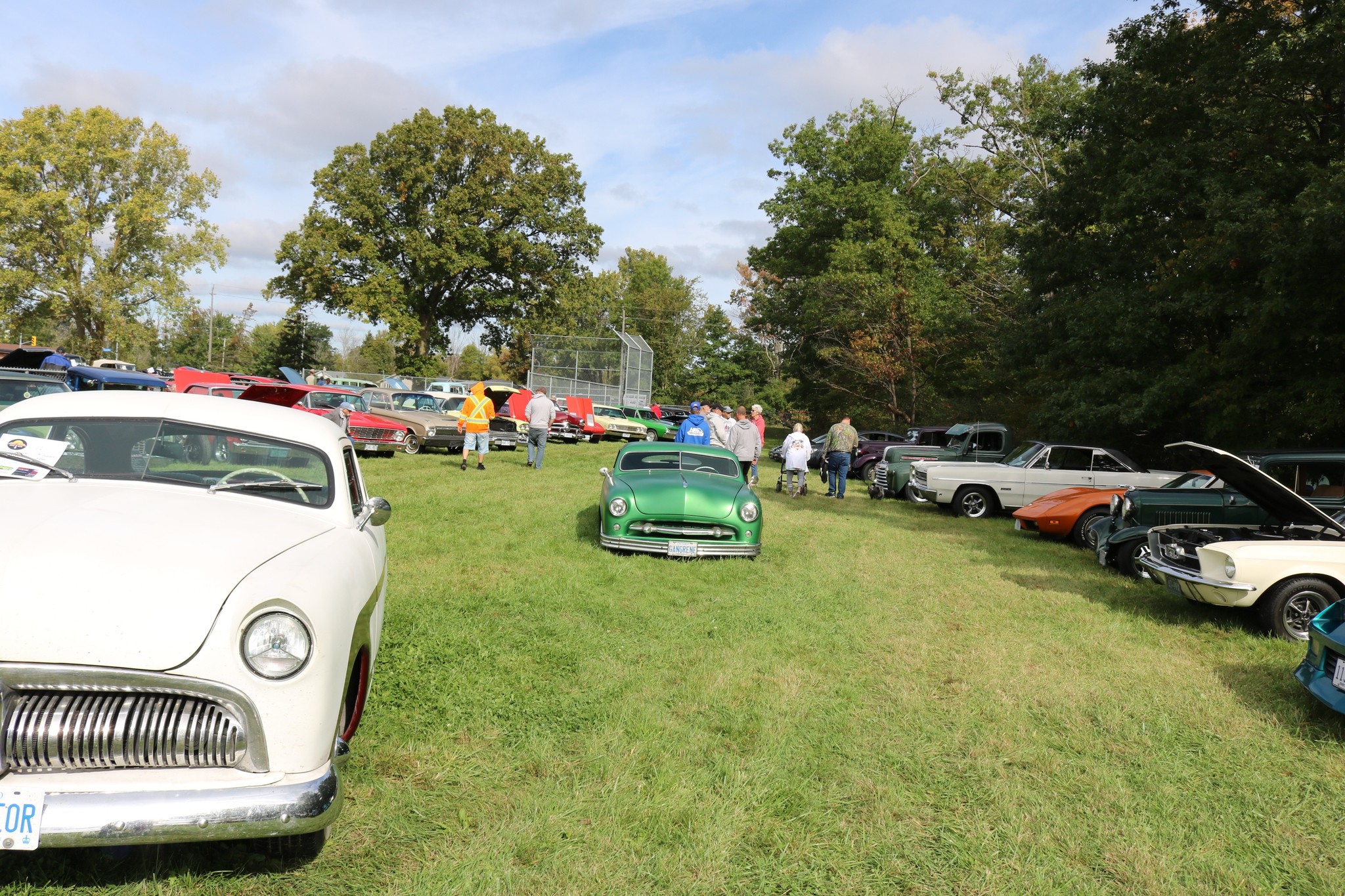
[752,410,757,412]
[727,412,732,413]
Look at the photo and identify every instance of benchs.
[124,470,327,507]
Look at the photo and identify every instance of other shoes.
[792,490,801,499]
[477,465,485,470]
[837,495,844,499]
[526,462,533,468]
[461,463,467,470]
[825,491,836,498]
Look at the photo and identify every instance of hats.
[712,403,726,410]
[723,406,733,413]
[690,402,702,411]
[340,402,354,411]
[700,401,713,406]
[56,346,65,353]
[310,369,317,373]
[535,386,547,394]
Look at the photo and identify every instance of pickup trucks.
[870,422,1010,505]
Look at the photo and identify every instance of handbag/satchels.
[819,459,827,484]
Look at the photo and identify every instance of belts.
[832,451,849,453]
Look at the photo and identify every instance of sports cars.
[1011,469,1233,552]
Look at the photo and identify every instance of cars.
[658,407,690,418]
[399,385,522,451]
[497,389,551,443]
[589,403,648,443]
[273,366,408,459]
[767,430,906,470]
[0,346,311,422]
[543,399,584,443]
[598,443,766,561]
[1139,441,1345,646]
[642,406,689,429]
[0,392,391,852]
[352,387,468,454]
[908,441,1225,518]
[562,396,605,443]
[1084,451,1345,581]
[597,405,679,444]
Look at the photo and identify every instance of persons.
[306,369,332,386]
[781,423,812,499]
[644,402,663,419]
[821,416,858,499]
[674,400,765,487]
[525,386,556,470]
[457,382,496,471]
[321,402,354,446]
